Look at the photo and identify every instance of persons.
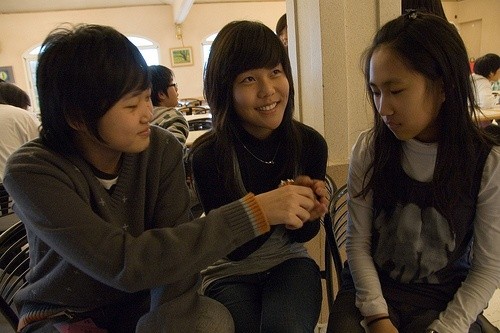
[0,82,43,280]
[326,12,500,333]
[3,24,315,333]
[276,13,288,49]
[467,53,500,147]
[148,66,201,210]
[190,20,329,333]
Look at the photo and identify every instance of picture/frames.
[169,46,194,67]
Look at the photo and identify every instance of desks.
[184,129,210,147]
[467,102,500,122]
[183,112,213,123]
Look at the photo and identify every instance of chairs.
[175,97,213,131]
[305,174,349,333]
[0,182,32,333]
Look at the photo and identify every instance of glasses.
[169,82,178,88]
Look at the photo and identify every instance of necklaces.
[238,137,281,164]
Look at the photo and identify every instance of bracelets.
[367,316,391,327]
[279,179,294,187]
